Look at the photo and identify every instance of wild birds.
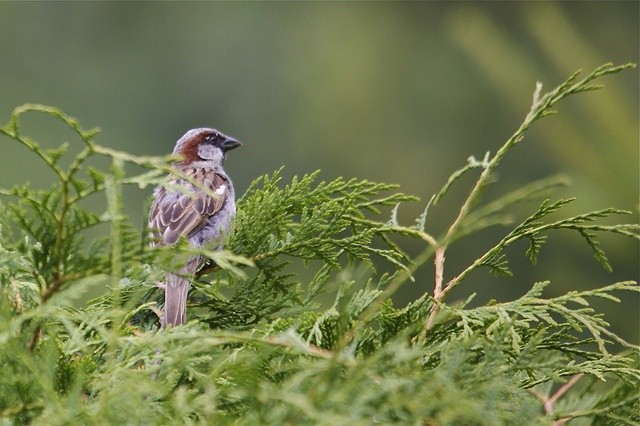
[147,127,243,331]
